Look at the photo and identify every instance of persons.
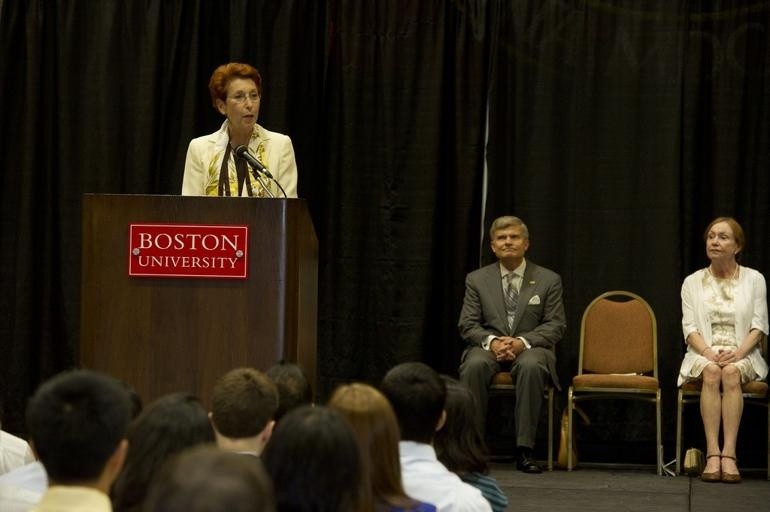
[678,216,769,484]
[180,64,298,198]
[458,216,567,475]
[1,366,510,511]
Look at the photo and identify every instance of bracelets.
[700,347,712,355]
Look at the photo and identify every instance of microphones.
[233,145,274,179]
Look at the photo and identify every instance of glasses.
[226,90,260,104]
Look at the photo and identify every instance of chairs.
[568,291,661,477]
[675,334,769,475]
[460,370,556,469]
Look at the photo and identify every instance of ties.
[506,273,518,328]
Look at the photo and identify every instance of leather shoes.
[516,456,541,474]
[701,454,721,482]
[720,455,742,483]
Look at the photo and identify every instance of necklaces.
[708,264,739,282]
